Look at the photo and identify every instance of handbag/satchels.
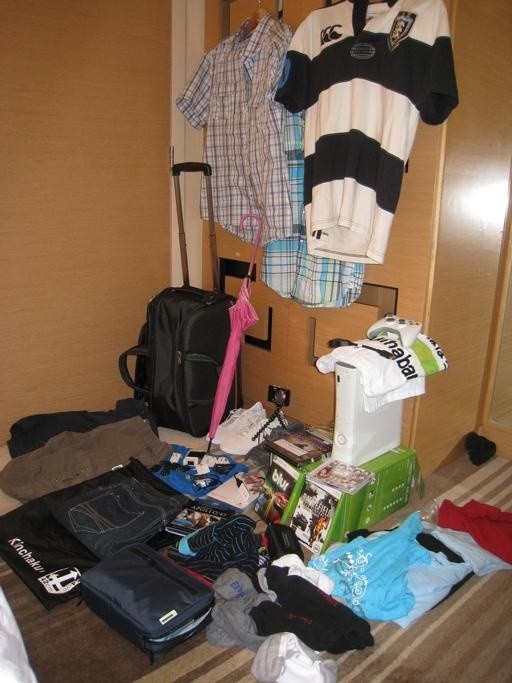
[78,542,217,665]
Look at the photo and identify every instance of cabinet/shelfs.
[201,0,512,493]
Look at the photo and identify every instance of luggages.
[118,161,243,438]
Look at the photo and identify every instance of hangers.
[240,1,271,38]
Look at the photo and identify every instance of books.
[207,428,375,558]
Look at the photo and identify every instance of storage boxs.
[292,447,426,555]
